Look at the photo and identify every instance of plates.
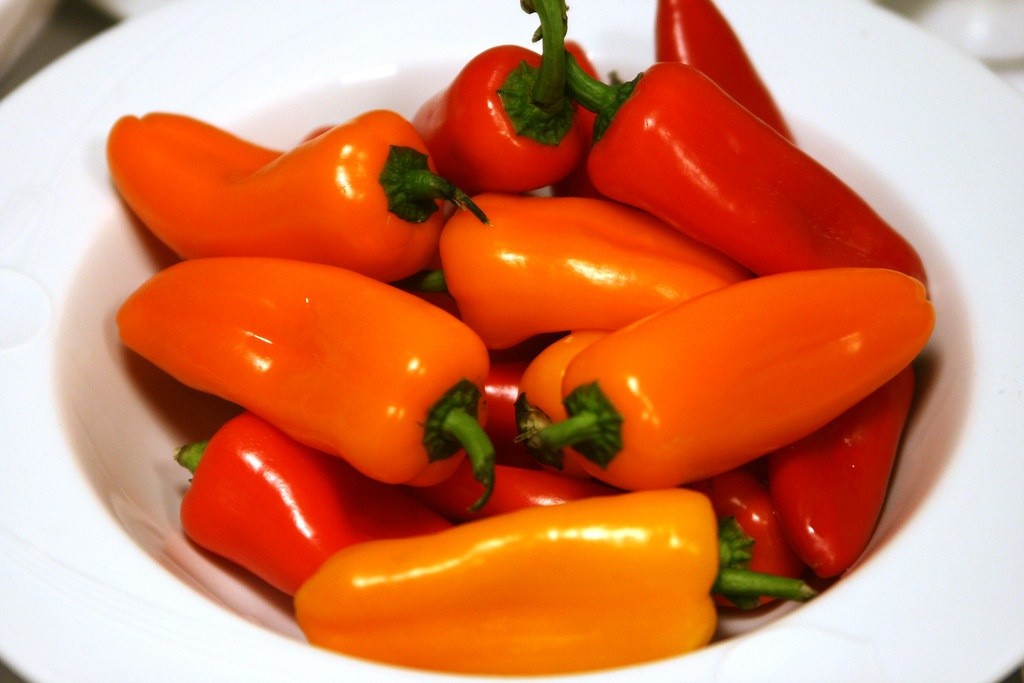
[0,0,1024,683]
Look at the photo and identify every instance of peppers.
[104,0,935,676]
